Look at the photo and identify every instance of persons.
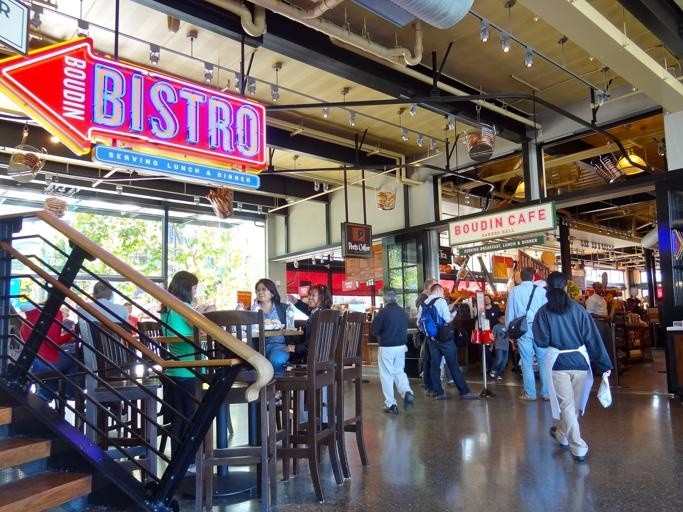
[475,295,510,380]
[533,272,612,463]
[20,280,138,401]
[157,270,203,470]
[505,270,550,399]
[248,278,333,368]
[370,291,414,412]
[587,282,612,363]
[416,279,481,399]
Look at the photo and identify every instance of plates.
[254,324,285,331]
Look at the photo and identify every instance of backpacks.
[419,298,443,338]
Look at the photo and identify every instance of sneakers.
[520,393,537,401]
[379,404,397,414]
[404,392,414,410]
[463,392,480,399]
[550,426,570,449]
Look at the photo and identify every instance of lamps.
[617,148,648,177]
[547,233,616,252]
[0,163,374,221]
[17,1,450,156]
[399,92,501,138]
[514,179,525,199]
[465,9,611,108]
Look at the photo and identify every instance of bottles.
[250,299,259,313]
[235,302,249,334]
[285,303,295,330]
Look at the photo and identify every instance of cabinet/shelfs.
[606,293,654,366]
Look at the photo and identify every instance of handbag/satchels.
[509,315,528,339]
[438,326,453,341]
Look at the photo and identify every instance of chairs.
[270,309,368,504]
[88,307,271,510]
